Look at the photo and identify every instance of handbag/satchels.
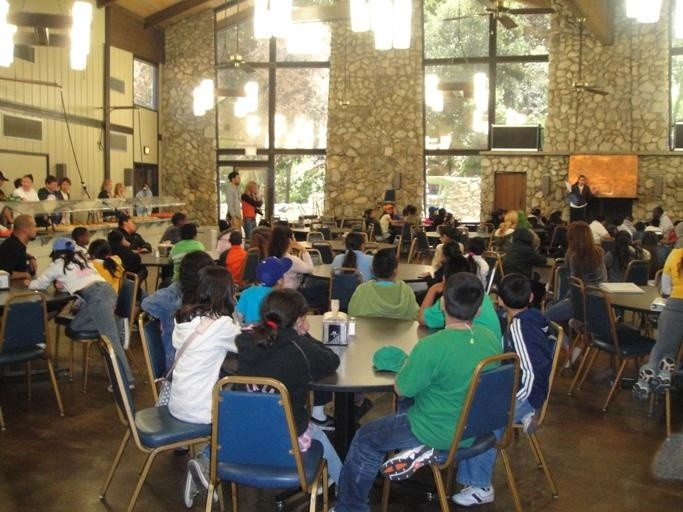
[155,314,222,407]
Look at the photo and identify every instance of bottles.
[348,318,357,336]
[322,300,346,347]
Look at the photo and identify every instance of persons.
[419,258,505,351]
[428,205,438,224]
[218,232,250,290]
[37,175,58,201]
[138,251,218,404]
[613,213,637,237]
[0,214,39,280]
[245,220,274,260]
[494,210,521,262]
[11,174,41,203]
[24,236,137,391]
[404,206,423,237]
[435,207,446,233]
[444,213,458,234]
[52,176,71,202]
[543,220,610,369]
[451,273,553,506]
[234,255,287,323]
[347,249,421,325]
[528,207,550,253]
[267,226,315,295]
[0,170,9,202]
[168,223,206,284]
[587,211,616,255]
[380,203,400,245]
[634,221,647,236]
[234,288,345,496]
[323,271,504,512]
[603,230,632,325]
[504,224,557,311]
[482,207,507,234]
[432,224,467,273]
[417,239,479,286]
[567,173,600,226]
[85,238,124,294]
[225,171,242,233]
[240,180,264,244]
[651,204,676,244]
[167,264,246,512]
[13,177,23,187]
[108,230,150,288]
[327,233,375,284]
[111,215,153,254]
[97,178,113,200]
[462,236,489,292]
[70,224,91,257]
[545,209,572,252]
[633,247,683,404]
[156,210,186,250]
[362,208,385,240]
[113,182,126,199]
[640,229,664,282]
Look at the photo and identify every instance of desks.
[310,263,436,283]
[424,231,490,239]
[134,248,220,292]
[298,241,397,253]
[222,315,444,512]
[292,227,352,234]
[589,282,670,389]
[0,278,77,378]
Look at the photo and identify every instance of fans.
[549,20,609,97]
[338,1,351,109]
[227,1,256,75]
[438,1,475,100]
[443,0,555,30]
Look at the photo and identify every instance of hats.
[373,346,408,373]
[49,239,75,257]
[383,205,393,210]
[256,256,293,283]
[269,227,294,242]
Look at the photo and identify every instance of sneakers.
[188,457,218,502]
[379,444,440,480]
[632,369,656,401]
[107,382,135,392]
[521,406,540,434]
[184,471,201,507]
[118,318,130,349]
[564,347,583,368]
[653,358,674,394]
[451,485,495,505]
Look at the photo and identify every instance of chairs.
[558,277,640,390]
[138,312,165,401]
[488,240,497,256]
[155,243,174,291]
[655,270,664,291]
[324,221,337,227]
[206,377,328,511]
[641,248,652,262]
[512,321,563,499]
[66,272,149,395]
[483,253,499,294]
[329,268,362,310]
[307,249,323,266]
[52,314,77,383]
[392,235,401,259]
[480,222,495,233]
[312,241,335,263]
[407,225,433,265]
[382,352,524,512]
[550,258,565,291]
[97,334,224,511]
[554,268,569,302]
[317,226,331,240]
[366,222,374,241]
[1,292,65,432]
[487,252,503,279]
[648,345,683,440]
[568,285,657,412]
[307,232,324,242]
[617,261,651,342]
[241,249,260,281]
[544,225,567,255]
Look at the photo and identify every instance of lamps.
[256,2,294,37]
[621,2,667,30]
[273,114,285,145]
[291,114,318,147]
[424,75,440,117]
[1,3,17,75]
[283,18,334,60]
[233,84,257,117]
[248,121,265,140]
[189,79,212,117]
[66,3,91,74]
[475,77,491,113]
[507,107,536,128]
